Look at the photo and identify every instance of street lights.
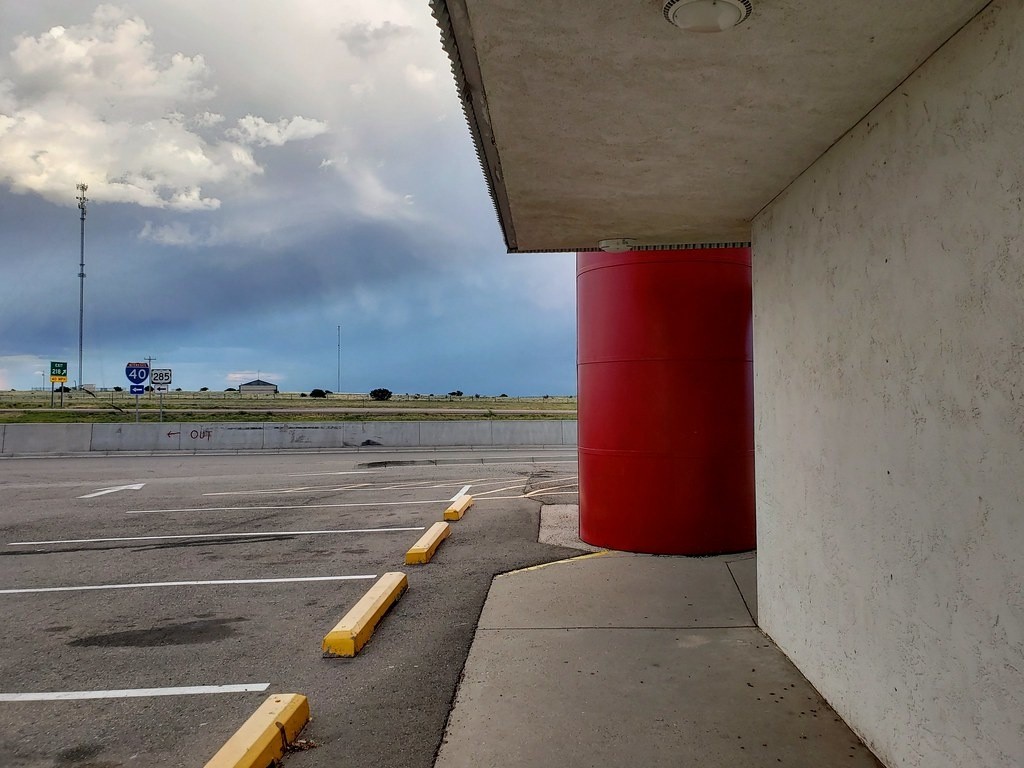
[75,182,90,389]
